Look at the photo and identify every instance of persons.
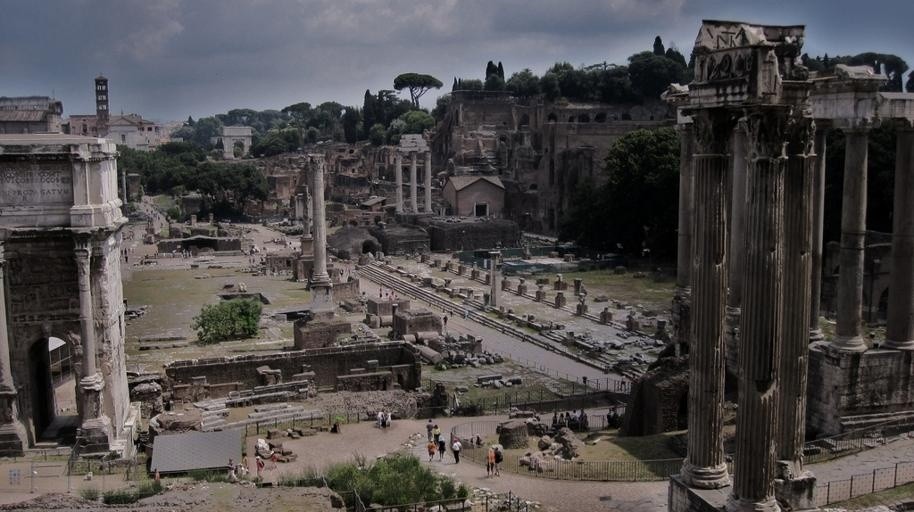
[256,456,265,480]
[452,439,462,463]
[236,463,244,480]
[426,419,445,462]
[620,373,637,391]
[377,409,391,428]
[380,288,398,300]
[270,451,280,474]
[154,468,159,480]
[242,453,249,475]
[226,459,237,479]
[443,315,448,325]
[552,408,587,432]
[486,447,501,478]
[464,309,468,320]
